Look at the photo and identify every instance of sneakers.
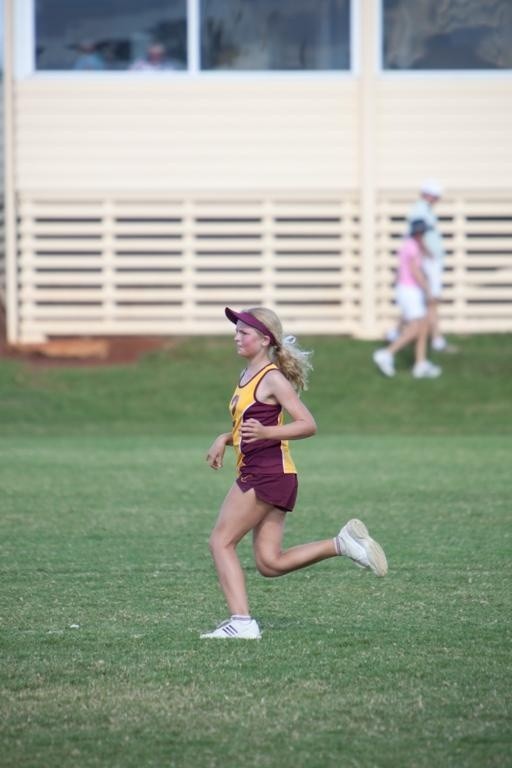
[433,344,461,355]
[413,361,443,380]
[199,618,261,641]
[374,348,395,378]
[337,518,388,579]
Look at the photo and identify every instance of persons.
[66,38,107,70]
[130,43,181,70]
[372,220,444,378]
[387,176,460,354]
[200,304,388,641]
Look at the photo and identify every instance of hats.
[421,183,444,197]
[224,308,277,346]
[412,222,434,235]
